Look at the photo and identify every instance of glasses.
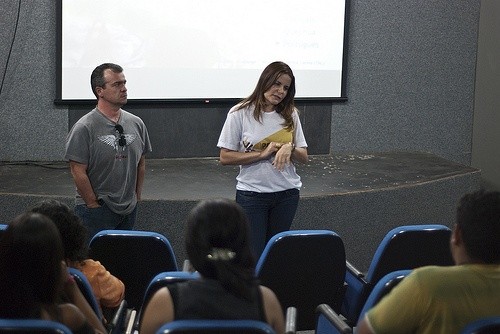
[115,124,126,146]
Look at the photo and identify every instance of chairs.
[0,226,500,334]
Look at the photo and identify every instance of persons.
[216,61,309,249]
[32,199,125,308]
[65,62,153,240]
[138,197,285,334]
[356,188,499,334]
[0,213,106,334]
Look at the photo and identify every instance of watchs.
[289,142,296,152]
